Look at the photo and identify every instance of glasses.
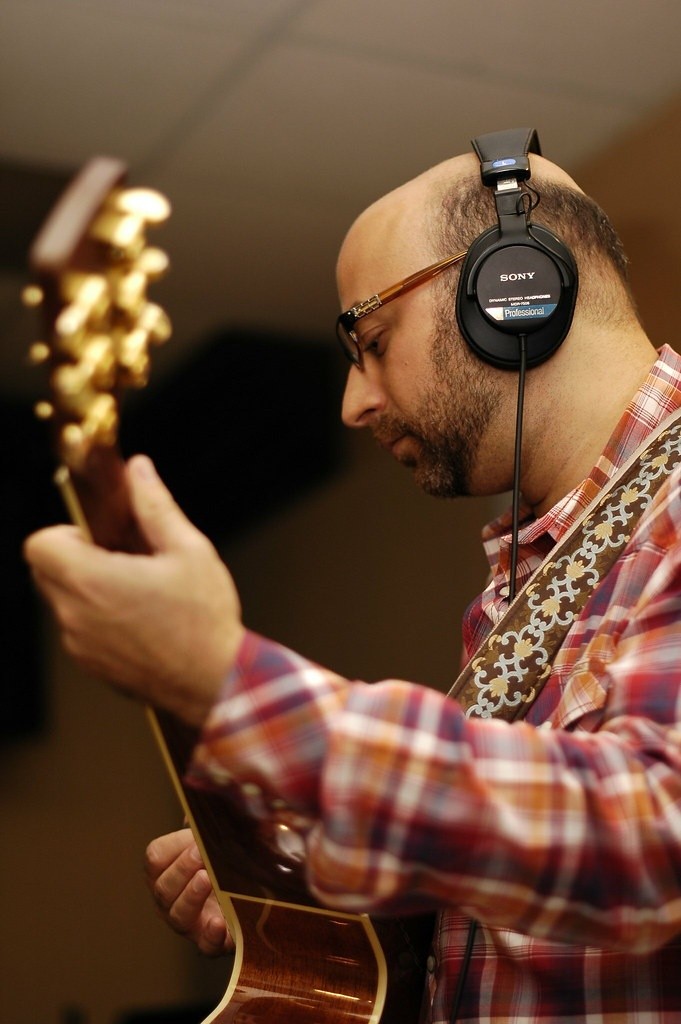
[335,251,470,374]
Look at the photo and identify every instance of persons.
[23,127,681,1024]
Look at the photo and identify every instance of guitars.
[22,153,439,1022]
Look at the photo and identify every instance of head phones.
[455,128,579,373]
